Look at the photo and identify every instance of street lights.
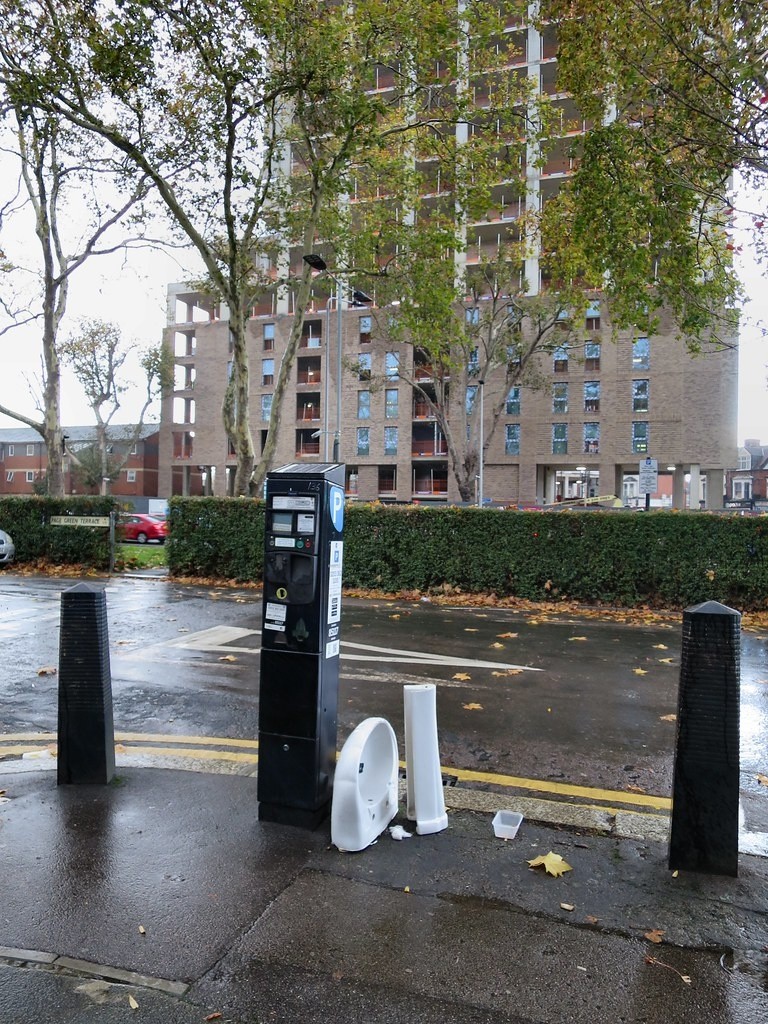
[302,256,373,465]
[324,297,366,462]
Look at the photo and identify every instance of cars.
[0,529,17,569]
[118,513,170,545]
